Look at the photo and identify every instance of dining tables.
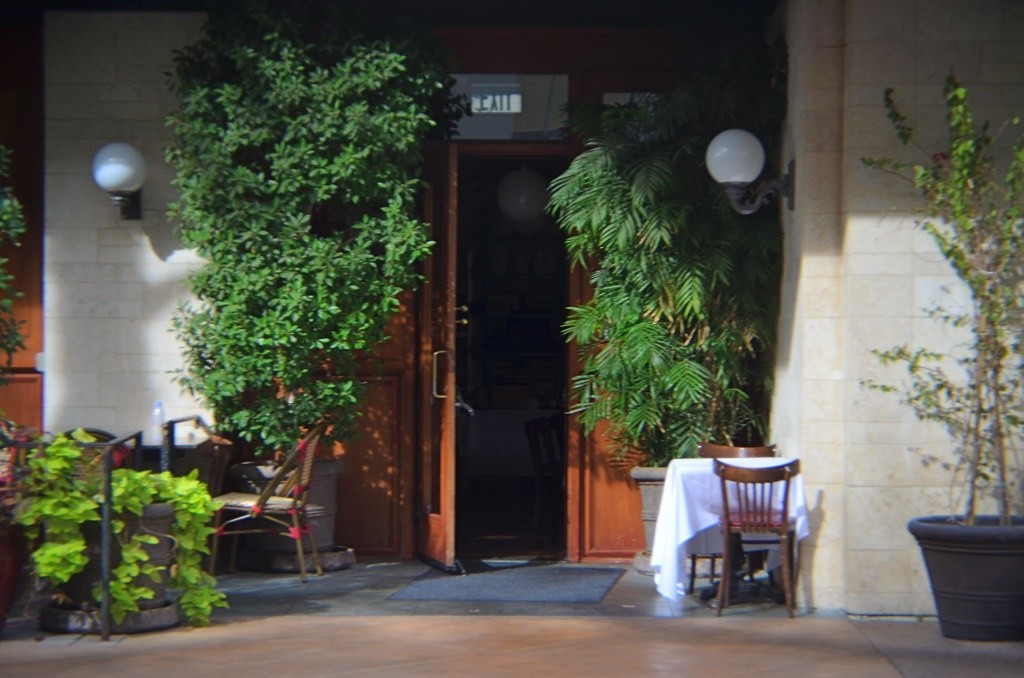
[669,457,802,603]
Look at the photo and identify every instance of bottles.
[151,402,165,445]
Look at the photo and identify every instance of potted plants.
[864,78,1024,642]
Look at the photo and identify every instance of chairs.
[525,412,565,533]
[209,421,323,582]
[712,458,800,618]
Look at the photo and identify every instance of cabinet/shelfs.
[466,240,567,408]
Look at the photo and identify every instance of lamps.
[706,128,794,215]
[93,141,146,219]
